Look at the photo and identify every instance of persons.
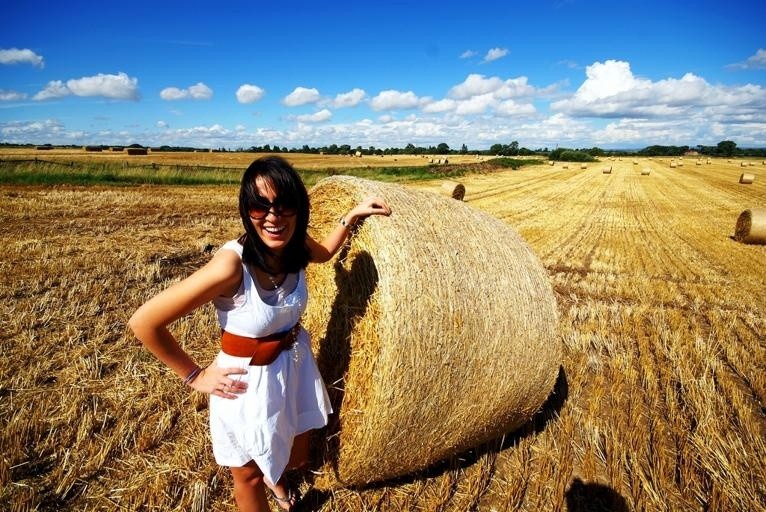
[128,155,392,512]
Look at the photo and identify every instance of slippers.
[263,473,297,512]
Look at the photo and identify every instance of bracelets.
[184,367,201,385]
[341,217,355,231]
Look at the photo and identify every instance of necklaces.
[268,274,302,362]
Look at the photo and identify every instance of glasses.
[246,201,298,219]
